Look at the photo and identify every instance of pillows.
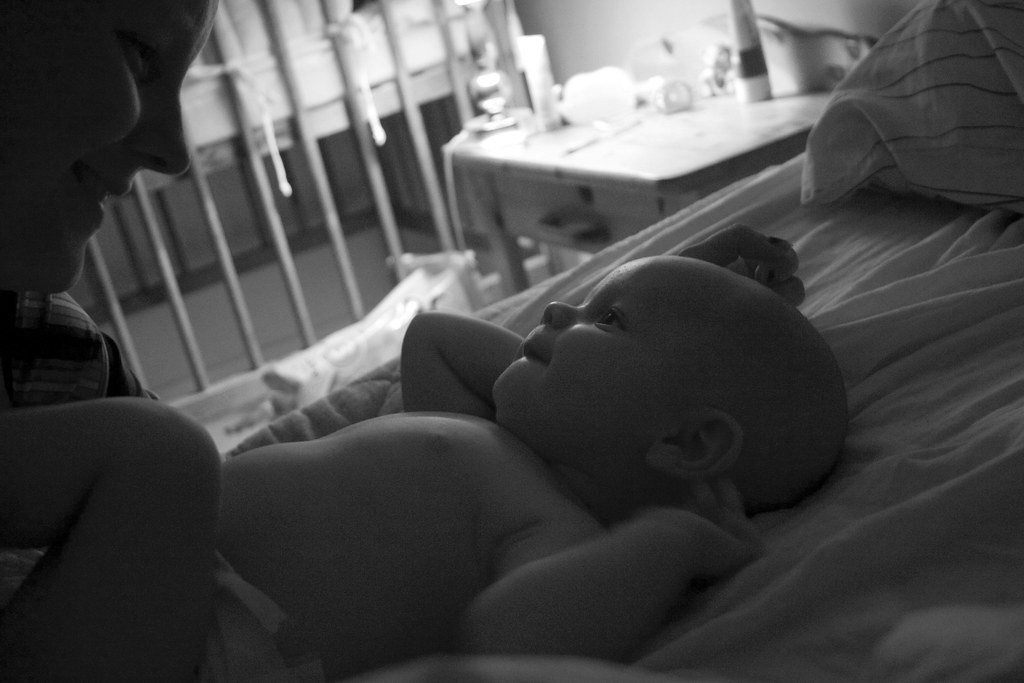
[799,0,1024,214]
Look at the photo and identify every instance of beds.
[286,148,1024,683]
[66,3,568,433]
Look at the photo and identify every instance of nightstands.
[445,84,833,303]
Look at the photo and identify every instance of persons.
[0,255,850,682]
[0,0,807,608]
